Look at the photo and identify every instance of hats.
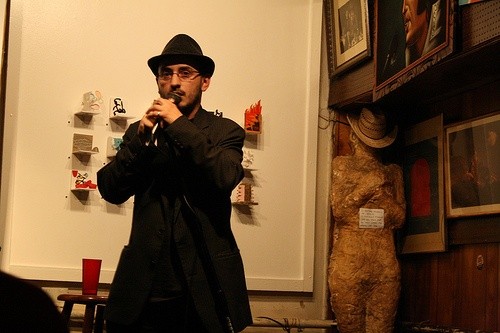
[347,106,399,148]
[148,33,215,80]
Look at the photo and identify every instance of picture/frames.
[443,111,500,218]
[326,0,372,79]
[372,0,459,101]
[390,116,448,254]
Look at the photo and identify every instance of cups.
[82,259,102,295]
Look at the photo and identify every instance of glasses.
[157,70,203,82]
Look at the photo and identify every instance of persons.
[328,131,405,333]
[97,35,253,333]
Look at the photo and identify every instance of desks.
[57,294,109,333]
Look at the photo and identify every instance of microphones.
[151,91,182,134]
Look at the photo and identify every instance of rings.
[157,111,160,116]
